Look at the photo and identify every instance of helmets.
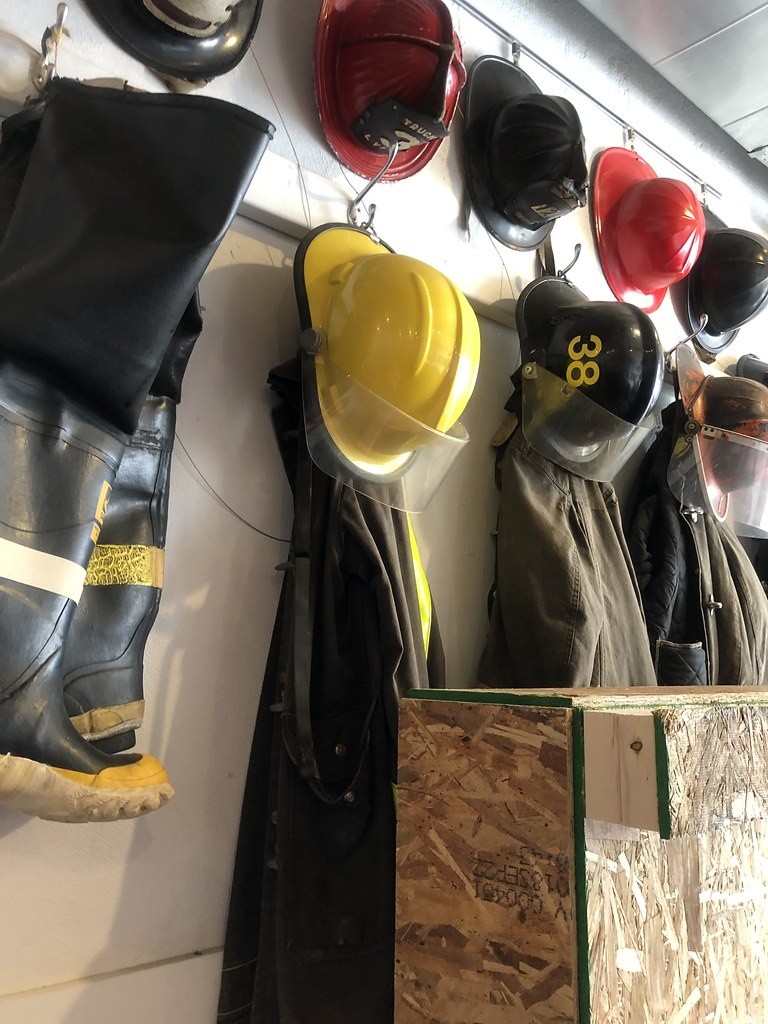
[294,221,482,484]
[676,343,768,522]
[515,276,665,463]
[737,355,768,390]
[595,147,706,315]
[686,202,768,353]
[313,0,467,187]
[466,54,592,253]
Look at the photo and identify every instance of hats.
[85,0,262,83]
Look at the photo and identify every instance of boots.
[3,74,279,825]
